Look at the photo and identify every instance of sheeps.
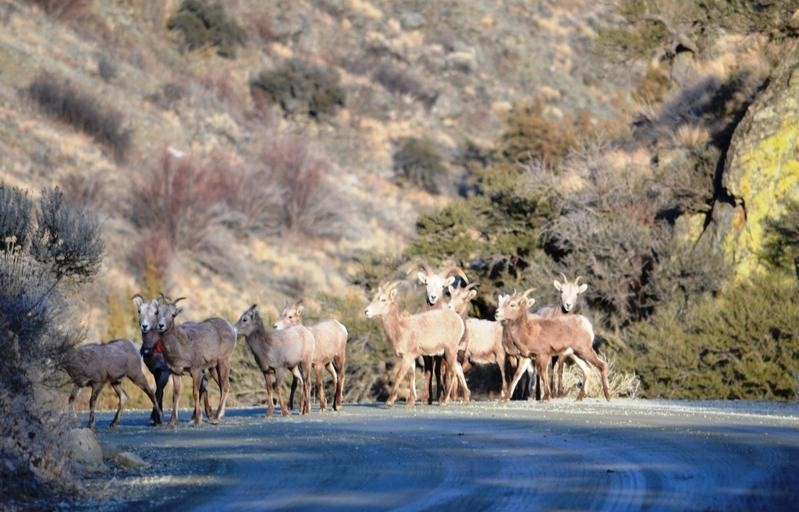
[416,259,611,404]
[231,302,316,417]
[273,298,350,411]
[44,338,166,432]
[130,293,215,425]
[155,291,239,432]
[364,280,472,406]
